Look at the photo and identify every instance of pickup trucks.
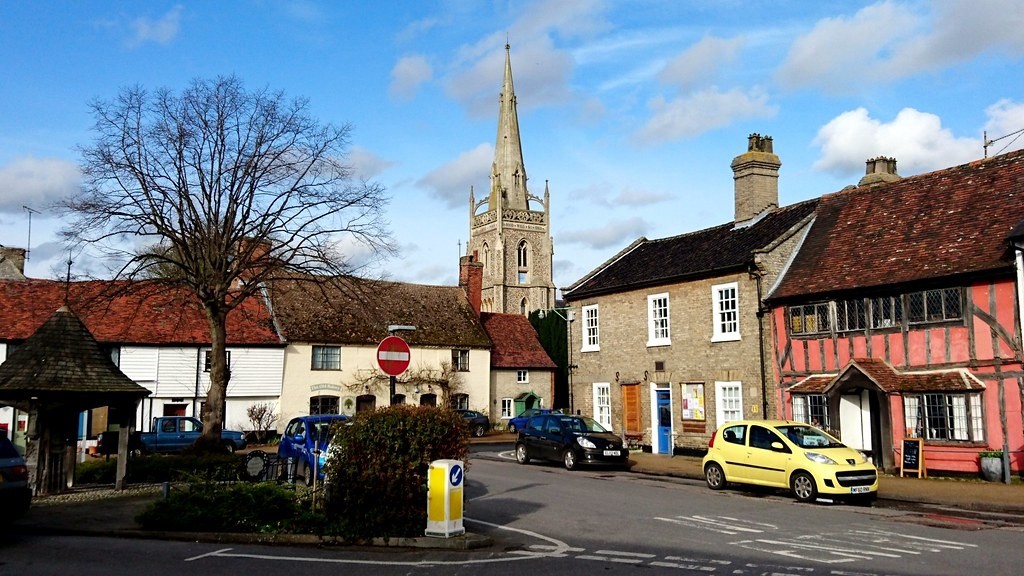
[97,415,248,460]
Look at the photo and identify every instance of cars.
[453,408,490,437]
[507,408,629,472]
[701,420,877,504]
[0,437,33,518]
[278,414,350,487]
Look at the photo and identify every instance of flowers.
[979,448,1003,458]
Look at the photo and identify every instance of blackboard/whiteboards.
[901,438,925,472]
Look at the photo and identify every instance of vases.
[980,457,1005,482]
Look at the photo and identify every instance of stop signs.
[376,336,410,376]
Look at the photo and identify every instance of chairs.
[293,424,330,441]
[725,429,803,449]
